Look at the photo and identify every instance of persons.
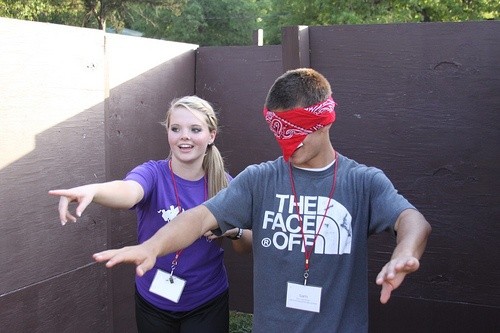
[93,67,432,333]
[48,95,252,333]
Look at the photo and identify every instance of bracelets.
[227,226,244,239]
